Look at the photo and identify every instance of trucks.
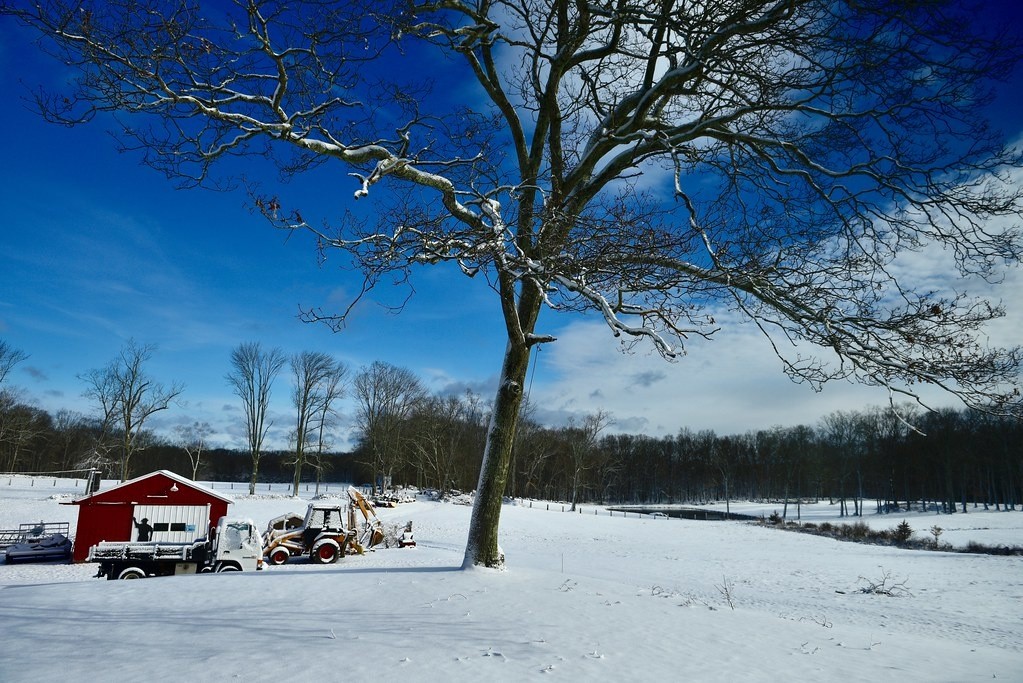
[85,516,265,580]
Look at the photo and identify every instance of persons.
[133,516,153,543]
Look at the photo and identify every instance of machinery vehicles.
[262,485,386,565]
